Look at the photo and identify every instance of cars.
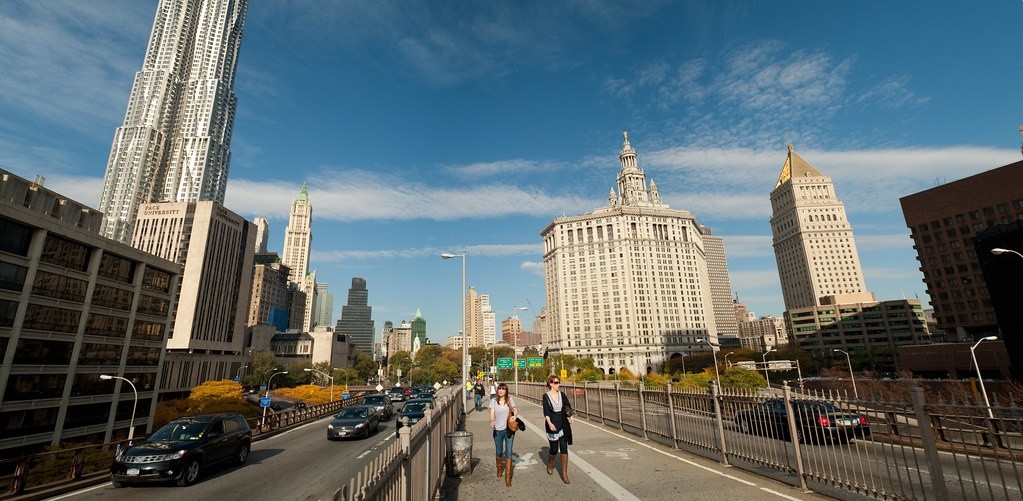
[396,399,435,438]
[416,394,437,406]
[735,398,870,444]
[327,405,379,440]
[405,386,436,396]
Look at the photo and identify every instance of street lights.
[697,339,723,400]
[682,354,686,377]
[725,351,733,370]
[833,349,859,400]
[398,357,409,382]
[441,251,467,415]
[261,371,288,428]
[512,306,528,398]
[100,374,137,446]
[970,336,998,418]
[333,367,348,390]
[762,348,777,386]
[236,365,249,379]
[303,368,333,404]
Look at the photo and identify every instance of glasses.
[498,388,505,390]
[553,381,560,385]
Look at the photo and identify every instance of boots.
[504,458,512,487]
[561,454,570,484]
[496,457,502,478]
[547,454,557,474]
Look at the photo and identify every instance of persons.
[543,375,577,485]
[473,379,497,412]
[466,379,472,400]
[488,383,518,487]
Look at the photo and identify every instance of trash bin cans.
[444,432,474,477]
[470,389,474,400]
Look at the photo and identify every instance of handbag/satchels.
[515,418,525,431]
[507,419,518,431]
[563,405,575,417]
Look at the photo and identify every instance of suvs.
[388,387,406,402]
[109,412,254,488]
[362,394,393,422]
